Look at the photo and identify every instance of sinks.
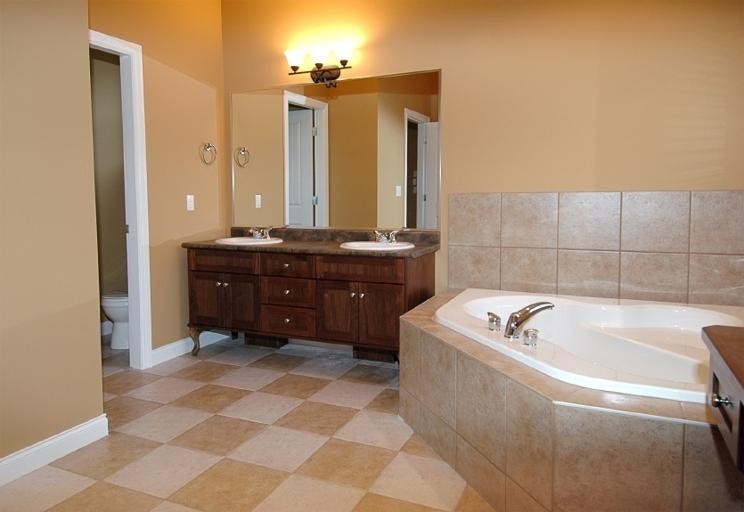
[215,236,284,245]
[339,240,415,251]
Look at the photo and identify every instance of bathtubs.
[430,286,743,405]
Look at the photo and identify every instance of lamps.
[282,38,362,91]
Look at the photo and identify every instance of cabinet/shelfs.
[315,253,435,363]
[259,252,315,341]
[186,247,258,358]
[704,348,744,479]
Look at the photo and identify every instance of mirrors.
[228,65,442,231]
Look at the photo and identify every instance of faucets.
[247,228,261,238]
[372,229,388,243]
[504,300,555,339]
